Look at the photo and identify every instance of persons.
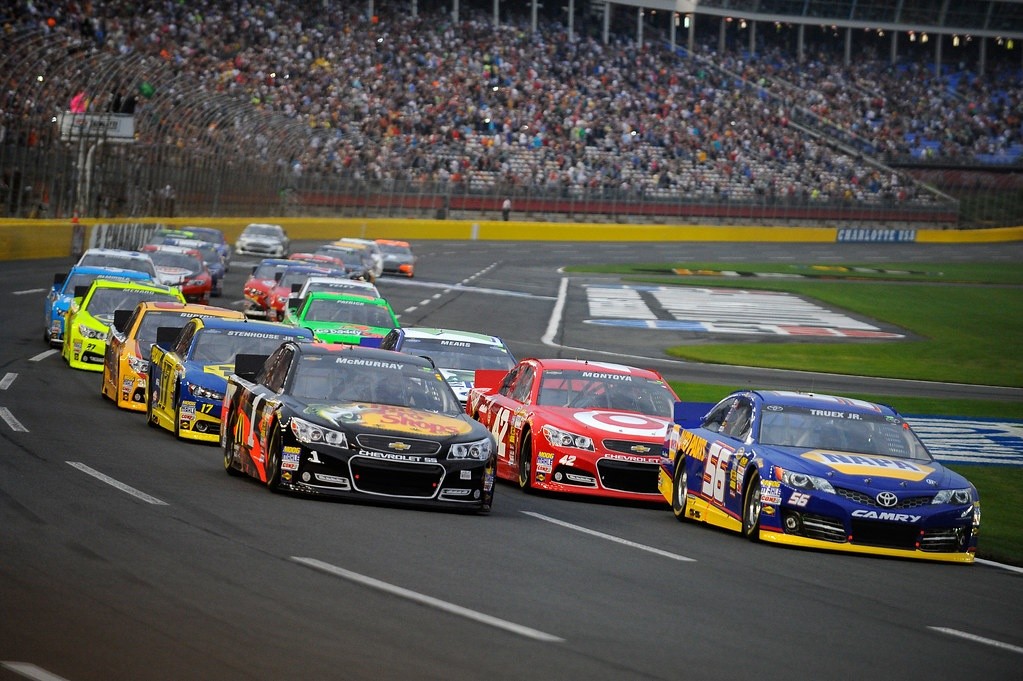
[0,0,1023,221]
[823,421,879,453]
[355,309,389,328]
[377,372,443,408]
[587,383,657,414]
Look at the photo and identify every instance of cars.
[61,278,187,374]
[219,340,498,515]
[467,357,683,501]
[282,290,401,346]
[76,249,161,286]
[314,237,384,284]
[658,389,982,563]
[141,244,212,306]
[243,258,318,311]
[360,326,519,413]
[287,254,344,270]
[44,266,152,343]
[283,276,380,316]
[147,227,234,298]
[266,266,342,322]
[235,224,291,260]
[102,301,248,415]
[376,239,417,278]
[148,316,322,444]
